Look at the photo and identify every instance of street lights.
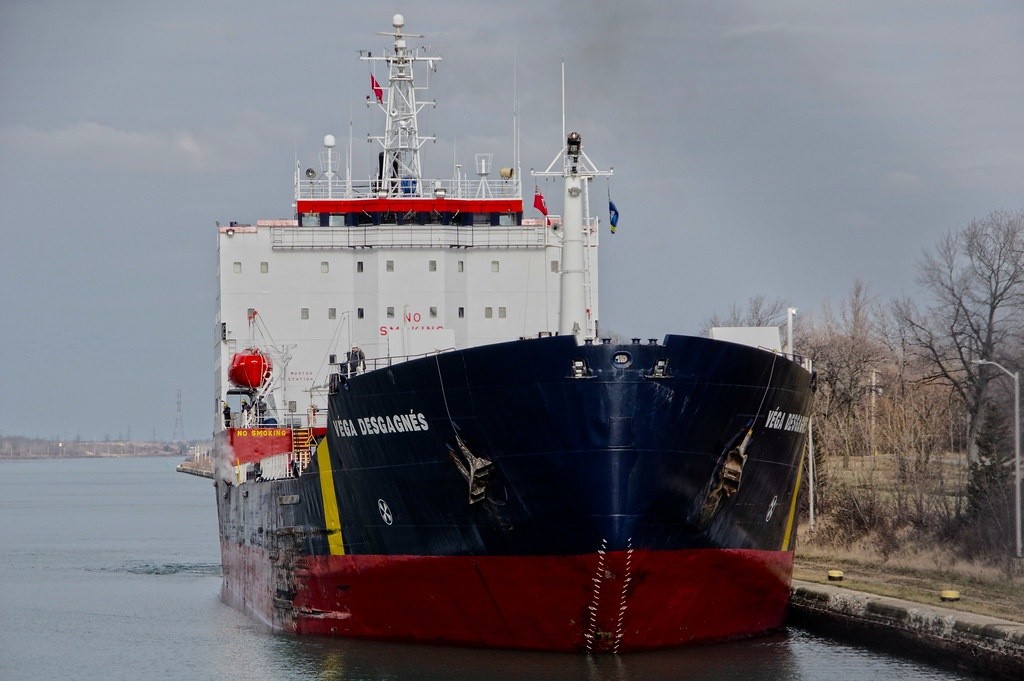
[972,359,1021,561]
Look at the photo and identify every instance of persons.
[241,399,251,413]
[352,346,366,373]
[223,404,231,427]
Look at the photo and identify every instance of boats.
[211,11,816,655]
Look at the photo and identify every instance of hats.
[242,400,246,403]
[223,404,228,407]
[351,343,358,348]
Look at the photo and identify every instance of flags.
[370,74,384,104]
[609,201,619,234]
[534,186,552,225]
